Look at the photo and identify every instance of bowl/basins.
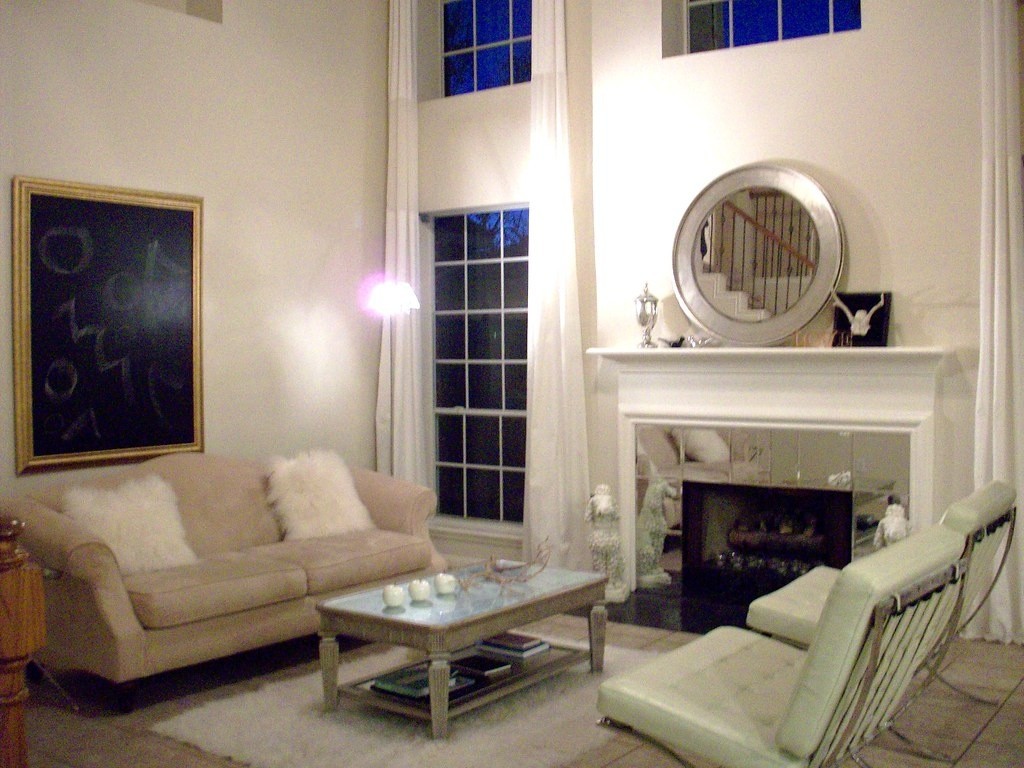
[409,578,430,601]
[382,584,404,607]
[435,572,457,595]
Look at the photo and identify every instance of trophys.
[635,282,658,349]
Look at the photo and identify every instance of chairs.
[747,479,1018,761]
[594,526,969,768]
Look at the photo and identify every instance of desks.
[584,344,959,592]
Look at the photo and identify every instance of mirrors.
[671,162,845,344]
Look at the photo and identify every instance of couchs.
[0,451,452,716]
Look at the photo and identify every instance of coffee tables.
[314,558,607,738]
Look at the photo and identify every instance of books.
[450,655,511,676]
[475,633,549,657]
[374,667,456,698]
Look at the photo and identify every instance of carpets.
[148,630,661,768]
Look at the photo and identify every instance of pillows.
[65,472,198,574]
[262,450,376,540]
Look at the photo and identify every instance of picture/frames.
[10,174,207,477]
